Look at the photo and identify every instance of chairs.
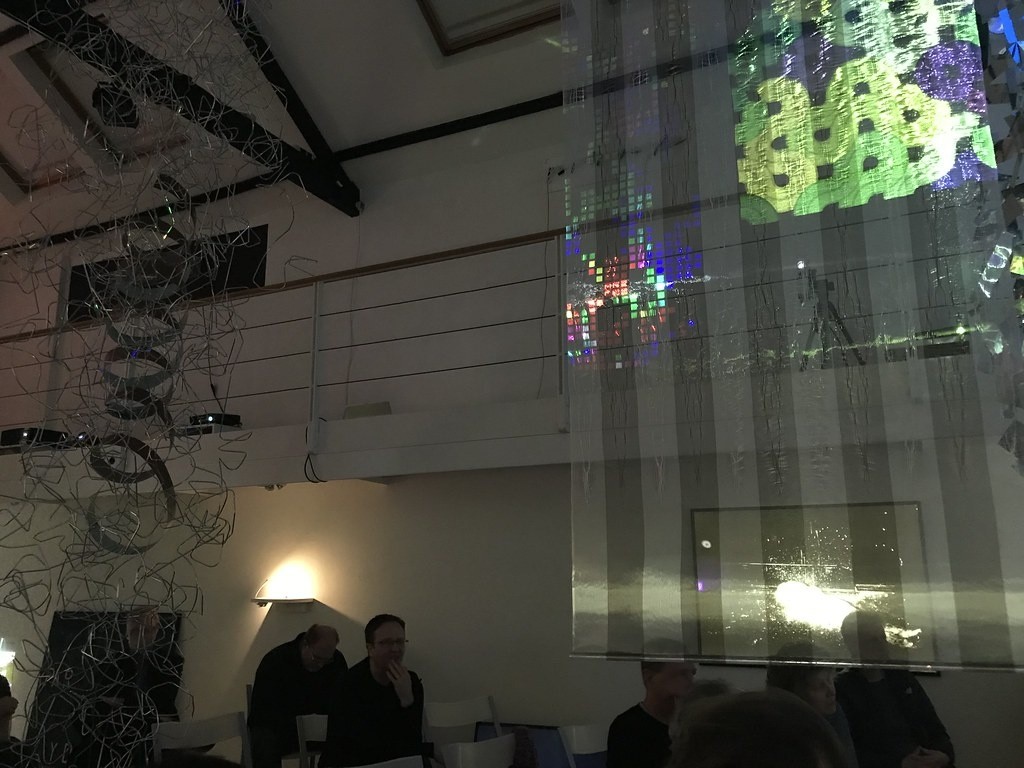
[151,683,609,768]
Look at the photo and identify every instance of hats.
[765,642,846,672]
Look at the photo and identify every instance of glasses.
[370,638,409,649]
[307,642,335,665]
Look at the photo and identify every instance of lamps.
[249,559,321,607]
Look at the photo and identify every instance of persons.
[318,614,424,768]
[608,613,955,768]
[249,625,348,768]
